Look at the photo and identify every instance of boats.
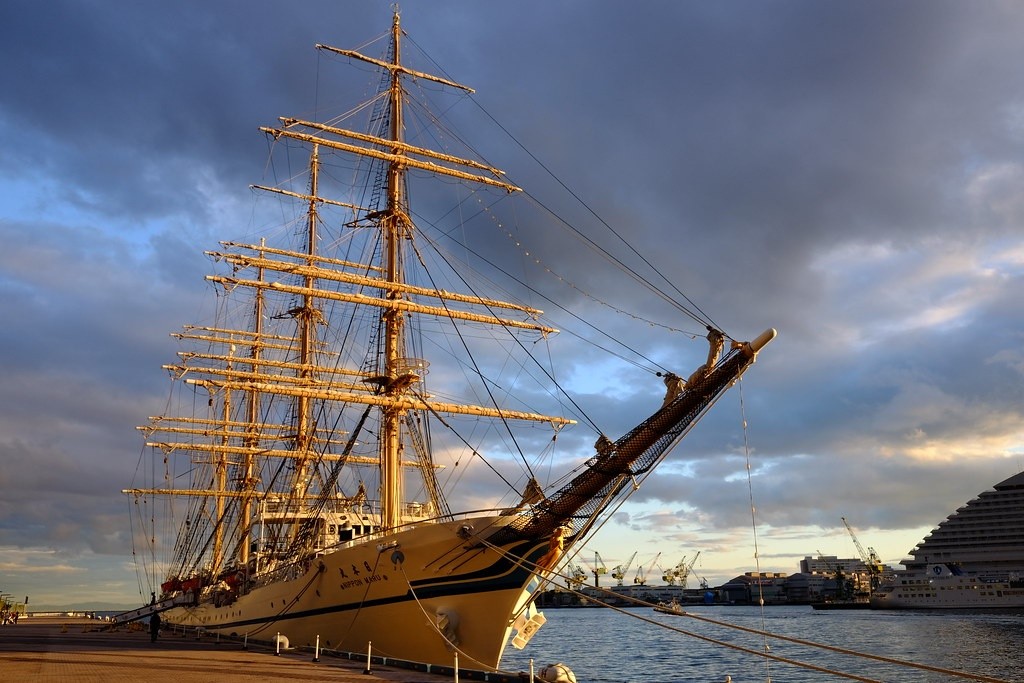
[653,596,686,615]
[810,517,1024,609]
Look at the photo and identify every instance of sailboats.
[121,2,778,671]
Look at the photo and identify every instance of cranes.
[837,517,885,577]
[557,545,700,591]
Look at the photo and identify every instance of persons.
[150,610,161,643]
[0,611,19,624]
